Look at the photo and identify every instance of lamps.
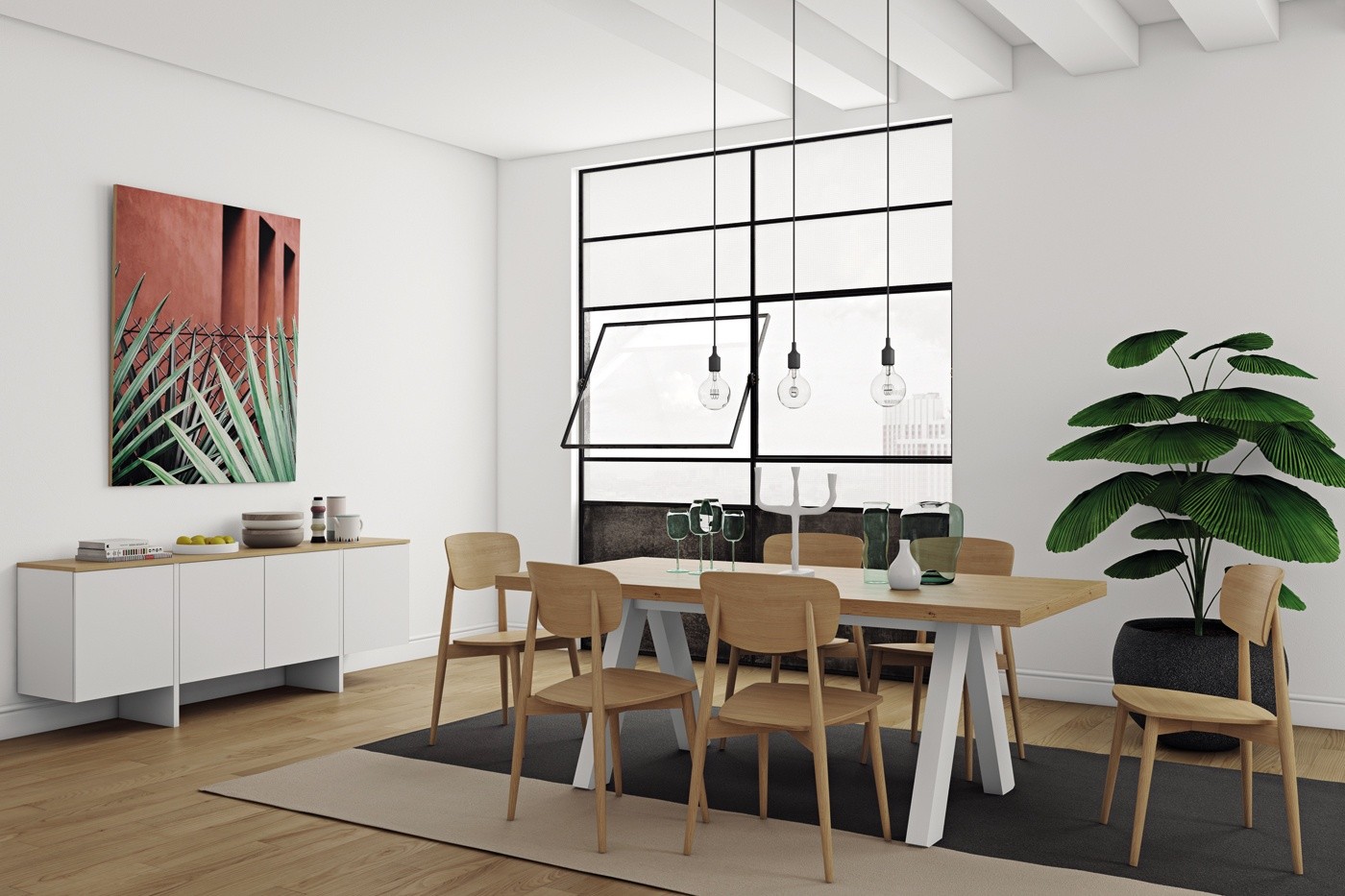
[696,0,732,409]
[775,0,811,409]
[872,0,906,407]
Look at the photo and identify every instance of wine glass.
[699,499,725,571]
[722,510,745,572]
[664,509,691,572]
[689,499,714,575]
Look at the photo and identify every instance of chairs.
[429,532,1026,884]
[1102,563,1305,878]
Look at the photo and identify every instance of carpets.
[198,707,1345,896]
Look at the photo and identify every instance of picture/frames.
[107,183,300,486]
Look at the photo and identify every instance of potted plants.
[1044,326,1345,749]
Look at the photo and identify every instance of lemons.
[177,535,235,545]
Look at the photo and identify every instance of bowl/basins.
[241,512,303,531]
[241,530,304,549]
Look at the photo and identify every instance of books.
[74,537,171,564]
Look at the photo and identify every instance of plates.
[173,542,240,554]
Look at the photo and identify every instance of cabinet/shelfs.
[17,538,413,728]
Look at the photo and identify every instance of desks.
[572,558,1107,843]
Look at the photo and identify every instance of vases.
[888,540,920,591]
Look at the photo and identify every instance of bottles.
[310,497,326,543]
[900,501,964,585]
[887,538,921,591]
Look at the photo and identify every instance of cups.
[334,515,363,541]
[862,501,890,585]
[326,496,347,541]
